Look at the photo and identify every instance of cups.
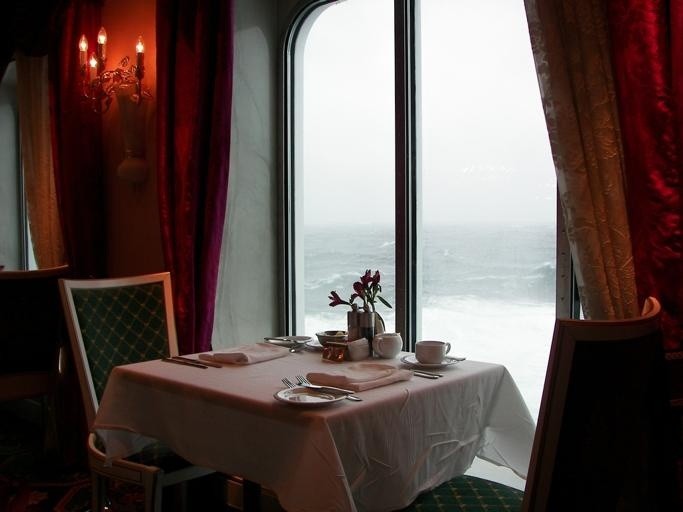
[371,331,403,359]
[347,337,369,361]
[414,341,451,364]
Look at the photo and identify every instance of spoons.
[263,336,291,341]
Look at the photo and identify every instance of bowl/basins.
[314,330,348,347]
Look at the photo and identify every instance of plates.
[400,354,464,369]
[273,386,348,405]
[266,336,312,346]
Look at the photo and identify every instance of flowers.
[325,265,391,311]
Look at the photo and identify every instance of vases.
[345,307,374,338]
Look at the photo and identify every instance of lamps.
[70,21,144,109]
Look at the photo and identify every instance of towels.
[304,358,414,390]
[194,340,291,366]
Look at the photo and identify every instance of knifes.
[161,356,208,369]
[408,367,442,379]
[171,355,221,367]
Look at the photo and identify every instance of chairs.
[50,269,244,512]
[0,264,69,465]
[400,295,666,512]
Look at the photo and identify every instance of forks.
[280,376,362,401]
[295,374,313,385]
